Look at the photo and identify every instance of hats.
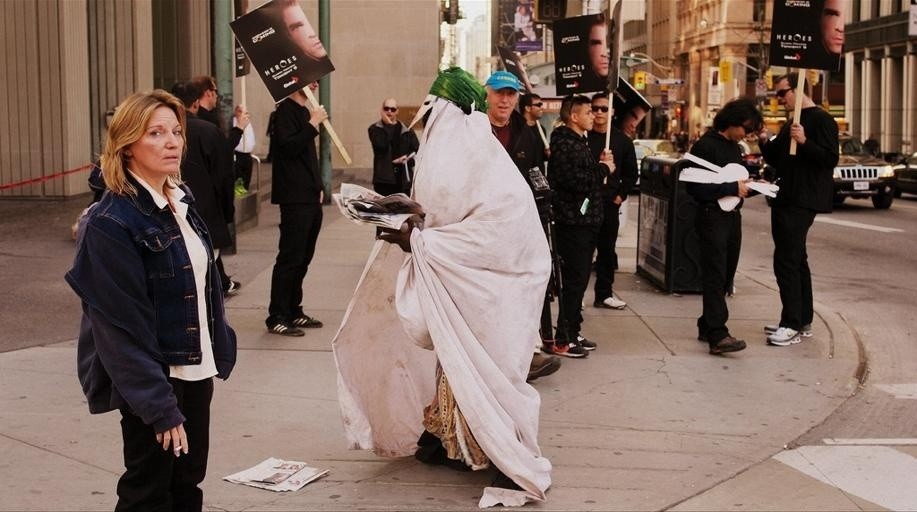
[486,71,521,92]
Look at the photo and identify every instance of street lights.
[630,52,674,136]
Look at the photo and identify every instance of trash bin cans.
[637,155,733,296]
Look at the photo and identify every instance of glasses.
[384,106,396,112]
[591,106,609,113]
[777,88,791,97]
[740,124,753,136]
[531,103,543,108]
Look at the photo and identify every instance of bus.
[529,83,603,181]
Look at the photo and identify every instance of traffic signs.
[654,78,685,85]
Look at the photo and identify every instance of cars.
[632,142,670,191]
[893,147,917,202]
[630,138,677,153]
[737,139,763,176]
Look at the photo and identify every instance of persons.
[367,66,639,493]
[514,4,537,43]
[801,0,846,73]
[670,72,839,355]
[660,109,670,139]
[63,74,250,512]
[263,75,330,336]
[275,0,336,82]
[575,20,608,92]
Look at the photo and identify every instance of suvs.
[757,131,894,212]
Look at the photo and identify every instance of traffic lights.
[675,105,682,119]
[636,72,645,89]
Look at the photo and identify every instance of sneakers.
[414,448,443,464]
[224,279,241,296]
[527,352,560,380]
[290,314,322,328]
[492,471,520,489]
[594,295,627,309]
[767,327,801,346]
[268,320,304,336]
[765,323,812,337]
[710,335,746,354]
[553,335,596,357]
